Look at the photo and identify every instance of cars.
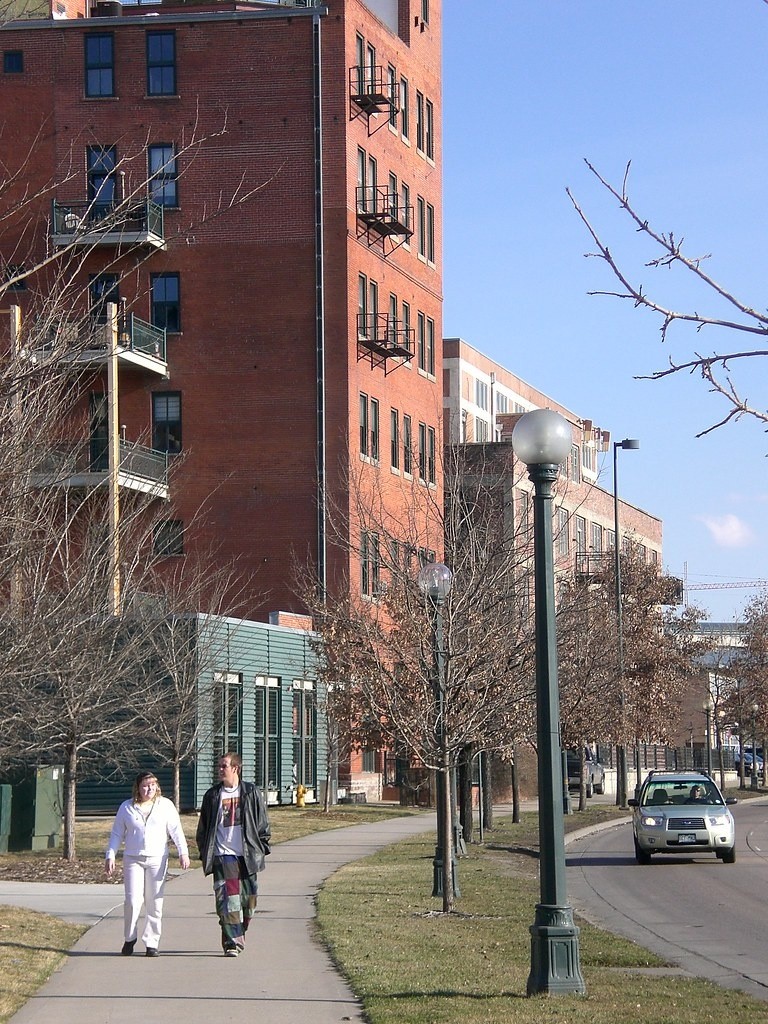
[734,753,764,777]
[563,743,606,798]
[627,767,738,864]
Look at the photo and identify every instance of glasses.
[218,765,231,769]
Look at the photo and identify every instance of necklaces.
[224,787,239,793]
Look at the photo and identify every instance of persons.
[684,785,713,804]
[105,770,190,956]
[196,753,270,957]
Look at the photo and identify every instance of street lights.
[508,409,587,995]
[612,439,641,808]
[748,702,760,792]
[702,699,715,780]
[421,563,461,900]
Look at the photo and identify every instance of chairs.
[63,320,80,349]
[650,789,669,805]
[64,215,86,233]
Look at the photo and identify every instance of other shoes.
[122,938,137,955]
[225,948,239,957]
[146,946,159,957]
